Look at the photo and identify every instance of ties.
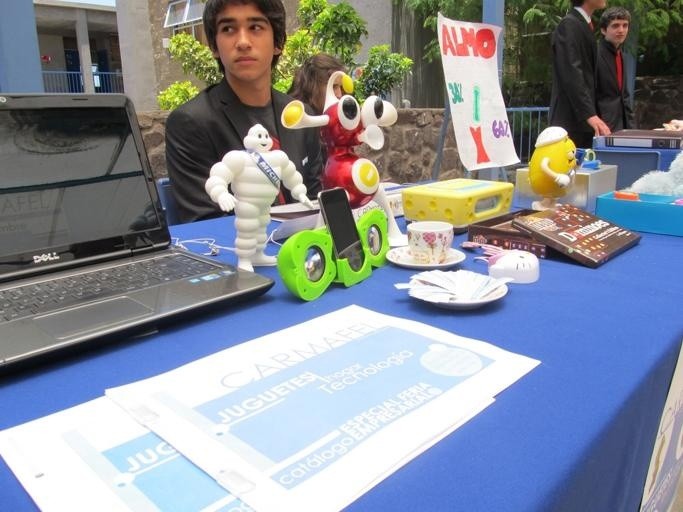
[589,22,595,33]
[615,48,623,91]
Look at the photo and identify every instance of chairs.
[156,177,180,226]
[585,147,660,190]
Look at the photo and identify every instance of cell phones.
[317,186,365,272]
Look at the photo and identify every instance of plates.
[409,273,508,310]
[385,245,466,270]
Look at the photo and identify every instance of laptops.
[0,92,275,368]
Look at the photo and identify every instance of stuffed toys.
[628,149,683,195]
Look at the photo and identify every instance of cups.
[406,221,453,264]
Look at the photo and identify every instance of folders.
[593,128,683,150]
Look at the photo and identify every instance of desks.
[0,181,683,512]
[591,143,683,190]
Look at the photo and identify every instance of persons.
[597,6,638,133]
[286,53,349,115]
[164,0,325,224]
[547,0,612,149]
